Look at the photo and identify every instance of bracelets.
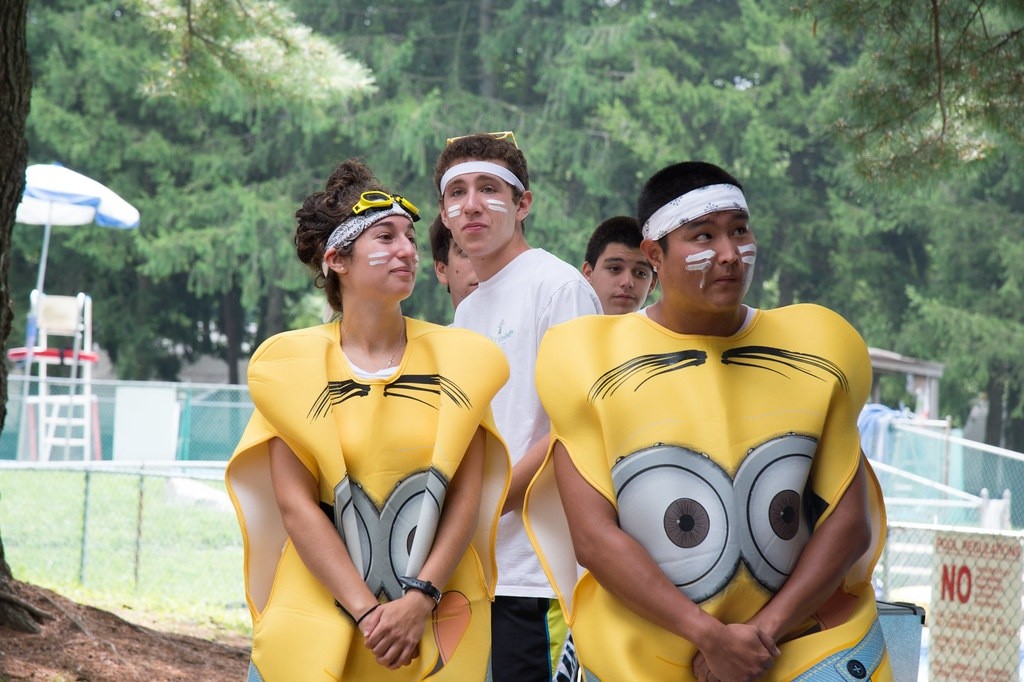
[356,604,382,627]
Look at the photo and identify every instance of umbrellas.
[15,163,142,460]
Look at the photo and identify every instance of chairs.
[8,291,98,371]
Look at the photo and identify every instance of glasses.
[446,131,519,149]
[352,190,421,222]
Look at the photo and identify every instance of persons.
[583,216,658,315]
[225,159,511,682]
[437,131,604,682]
[523,162,896,682]
[430,214,480,311]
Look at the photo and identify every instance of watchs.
[400,577,441,612]
